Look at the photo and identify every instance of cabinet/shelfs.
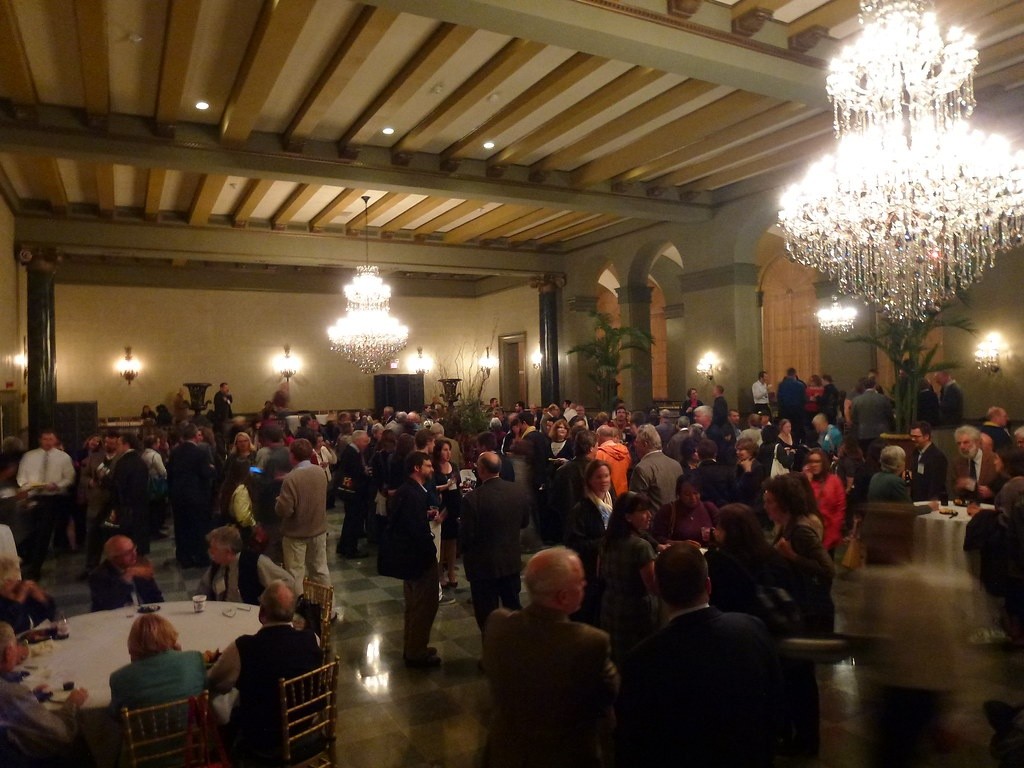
[56,401,98,464]
[374,372,424,418]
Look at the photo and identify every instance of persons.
[109,613,206,758]
[0,368,1024,625]
[613,543,792,768]
[203,582,326,768]
[477,548,619,768]
[763,476,834,754]
[0,624,88,768]
[388,453,445,671]
[837,547,956,768]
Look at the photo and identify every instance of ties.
[970,460,978,481]
[39,453,49,490]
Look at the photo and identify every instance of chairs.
[276,655,340,768]
[318,602,334,690]
[121,690,212,768]
[302,577,334,610]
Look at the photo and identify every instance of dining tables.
[907,501,997,567]
[10,599,264,768]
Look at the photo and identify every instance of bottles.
[708,527,716,551]
[57,611,69,639]
[907,468,911,495]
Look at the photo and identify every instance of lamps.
[974,335,1008,374]
[279,343,299,382]
[696,348,723,381]
[530,349,542,370]
[774,1,1024,327]
[813,294,858,335]
[414,345,429,376]
[118,344,141,383]
[324,196,409,375]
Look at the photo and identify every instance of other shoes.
[420,646,437,656]
[326,609,338,625]
[442,584,451,590]
[446,582,459,587]
[420,657,441,667]
[439,596,456,605]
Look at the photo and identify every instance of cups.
[63,668,74,690]
[125,602,135,618]
[193,595,207,612]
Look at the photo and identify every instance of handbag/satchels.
[186,693,235,768]
[770,444,790,480]
[292,595,323,639]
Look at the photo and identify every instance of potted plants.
[844,270,979,474]
[439,318,498,469]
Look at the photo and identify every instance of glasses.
[115,544,139,558]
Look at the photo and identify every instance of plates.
[138,605,161,613]
[50,689,73,701]
